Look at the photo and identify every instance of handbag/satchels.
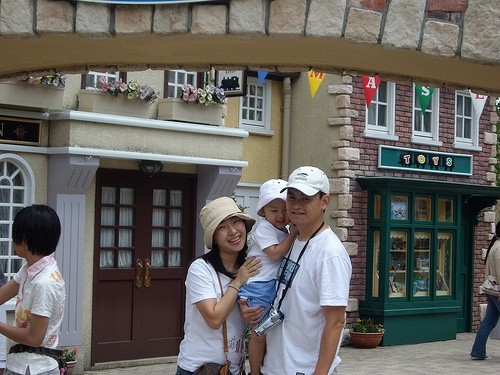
[9,344,68,375]
[193,360,246,375]
[481,275,500,296]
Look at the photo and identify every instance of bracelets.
[228,285,239,292]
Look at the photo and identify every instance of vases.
[78,90,150,119]
[155,97,223,125]
[65,361,76,375]
[0,81,62,112]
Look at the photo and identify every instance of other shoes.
[471,355,490,360]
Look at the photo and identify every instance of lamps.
[139,160,164,177]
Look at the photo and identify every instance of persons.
[0,205,67,375]
[176,197,263,375]
[240,166,353,375]
[237,179,298,375]
[469,221,500,359]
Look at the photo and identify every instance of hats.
[199,197,256,252]
[257,178,288,217]
[280,166,329,197]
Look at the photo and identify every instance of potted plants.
[349,319,384,348]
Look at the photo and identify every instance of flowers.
[181,84,228,105]
[22,72,66,90]
[59,348,77,362]
[99,77,160,101]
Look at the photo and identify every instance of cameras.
[255,308,285,336]
